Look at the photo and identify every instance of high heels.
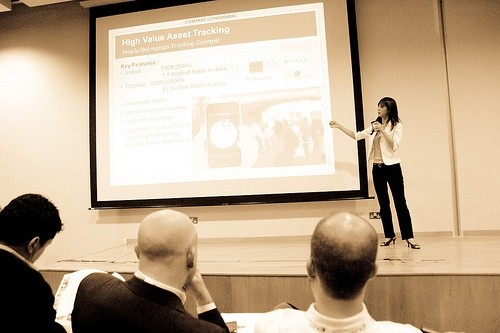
[380,236,396,246]
[407,238,420,249]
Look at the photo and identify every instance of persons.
[71,210,230,333]
[247,212,425,333]
[330,97,422,250]
[0,194,69,333]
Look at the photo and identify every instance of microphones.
[370,116,382,135]
